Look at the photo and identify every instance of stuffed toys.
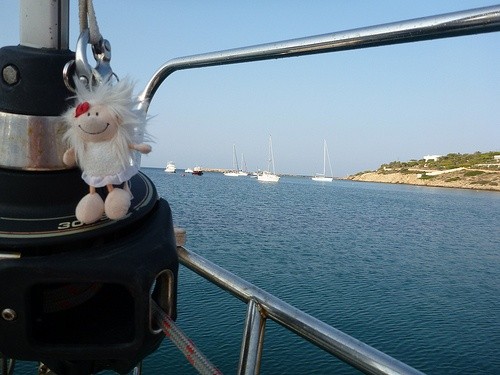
[58,73,158,223]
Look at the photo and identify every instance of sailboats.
[257,134,281,182]
[311,139,334,182]
[238,150,249,177]
[222,144,240,177]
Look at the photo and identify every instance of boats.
[164,161,176,173]
[191,166,203,176]
[185,168,193,173]
